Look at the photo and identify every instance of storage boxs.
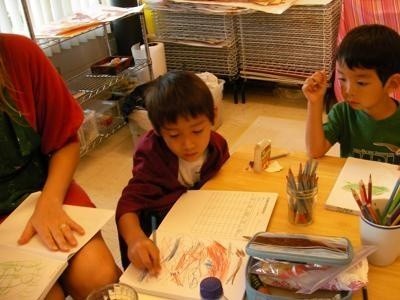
[88,101,121,135]
[209,79,226,127]
[76,108,101,148]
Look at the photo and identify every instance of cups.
[286,176,318,226]
[360,198,400,267]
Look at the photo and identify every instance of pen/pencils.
[362,286,368,300]
[151,216,158,279]
[250,154,288,165]
[351,173,400,226]
[285,159,319,224]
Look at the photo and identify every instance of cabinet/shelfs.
[40,12,152,155]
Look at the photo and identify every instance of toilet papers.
[130,42,168,79]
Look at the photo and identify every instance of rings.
[59,224,69,228]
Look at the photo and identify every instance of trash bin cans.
[195,78,225,131]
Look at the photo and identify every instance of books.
[119,188,278,300]
[326,157,400,217]
[0,191,116,300]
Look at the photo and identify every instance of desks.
[119,145,400,300]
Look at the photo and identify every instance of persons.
[115,71,230,271]
[302,24,400,166]
[0,32,124,300]
